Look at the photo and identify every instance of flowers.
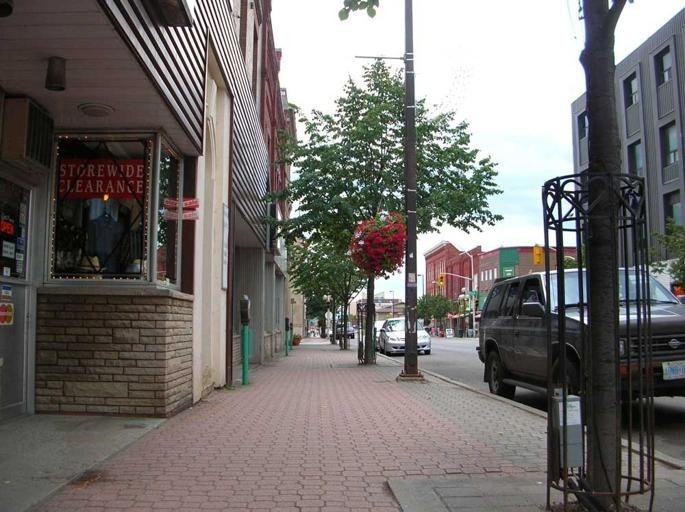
[348,208,408,279]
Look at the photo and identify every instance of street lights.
[432,240,476,337]
[387,273,424,316]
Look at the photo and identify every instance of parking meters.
[240,295,251,385]
[285,316,293,356]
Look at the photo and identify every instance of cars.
[334,321,355,339]
[476,266,685,425]
[372,317,431,357]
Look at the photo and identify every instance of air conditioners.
[1,95,55,177]
